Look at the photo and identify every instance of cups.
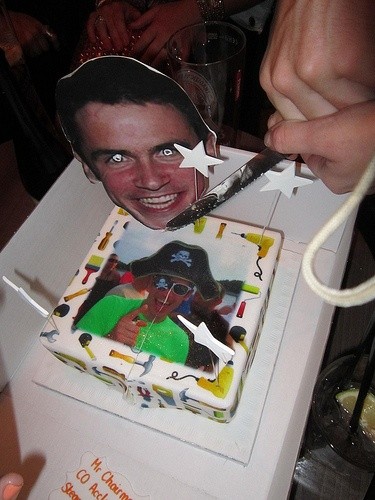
[165,21,245,149]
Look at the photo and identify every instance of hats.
[129,240,222,301]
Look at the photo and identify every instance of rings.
[95,14,105,25]
[42,24,59,42]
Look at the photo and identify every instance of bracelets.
[96,0,108,8]
[196,1,226,24]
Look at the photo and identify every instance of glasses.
[151,274,190,296]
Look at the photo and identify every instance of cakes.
[39,203,283,424]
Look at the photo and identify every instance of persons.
[54,54,218,230]
[100,254,122,282]
[70,0,259,76]
[259,0,374,198]
[1,0,97,128]
[75,239,219,365]
[169,280,230,373]
[103,256,151,301]
